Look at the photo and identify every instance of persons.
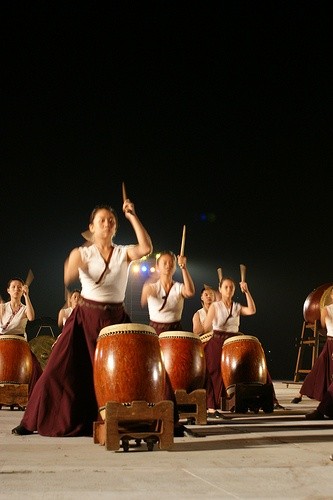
[11,201,180,437]
[193,286,217,338]
[0,278,43,408]
[299,286,333,422]
[59,289,80,329]
[141,251,194,334]
[203,279,285,413]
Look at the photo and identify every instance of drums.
[94,324,165,420]
[200,331,245,346]
[303,285,333,335]
[221,335,267,395]
[159,331,206,393]
[0,335,34,385]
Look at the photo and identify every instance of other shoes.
[307,409,324,419]
[11,425,34,435]
[292,397,302,403]
